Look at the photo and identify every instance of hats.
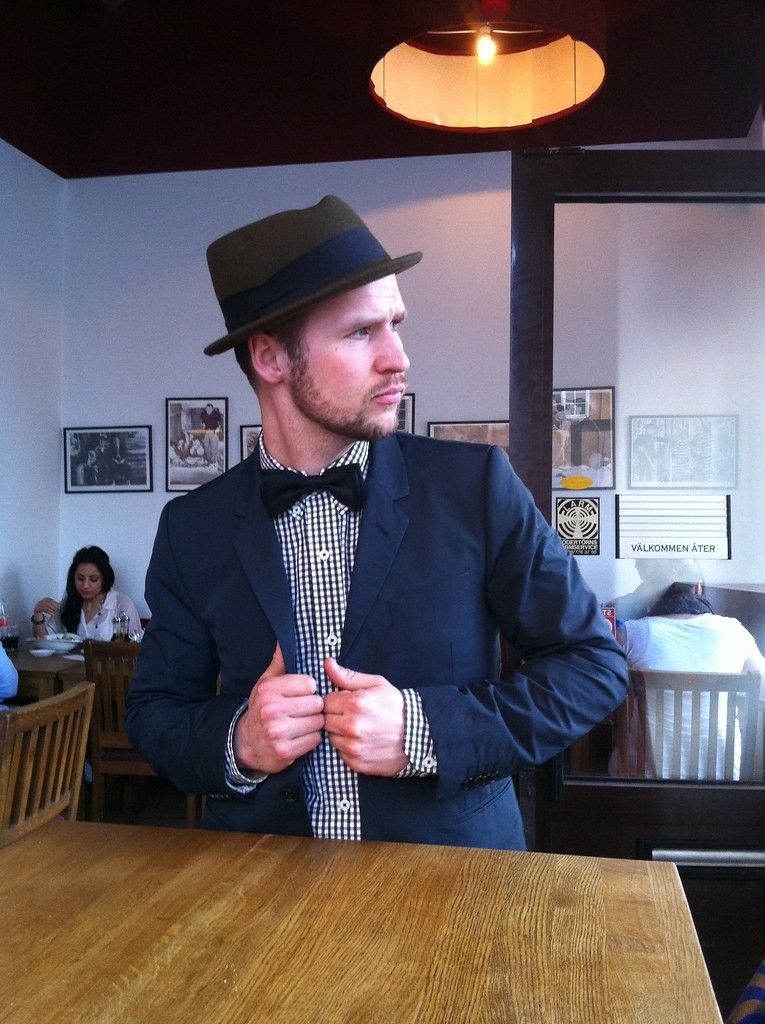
[203,195,423,356]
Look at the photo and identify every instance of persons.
[609,557,765,781]
[122,194,629,854]
[31,541,144,778]
[170,404,223,466]
[71,432,135,485]
[0,638,93,782]
[552,396,585,429]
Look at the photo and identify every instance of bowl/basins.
[32,639,79,654]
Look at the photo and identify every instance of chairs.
[618,667,760,782]
[1,681,94,847]
[82,636,197,828]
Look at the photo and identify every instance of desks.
[1,821,724,1023]
[5,647,85,704]
[59,660,221,698]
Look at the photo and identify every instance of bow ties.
[259,464,367,517]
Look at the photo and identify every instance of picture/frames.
[552,386,616,490]
[628,413,740,489]
[64,425,153,493]
[165,397,229,492]
[240,424,263,462]
[426,420,510,456]
[394,392,416,435]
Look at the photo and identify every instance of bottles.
[0,600,10,639]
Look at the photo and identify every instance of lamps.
[369,0,609,134]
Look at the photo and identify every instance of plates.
[29,649,55,658]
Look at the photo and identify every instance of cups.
[113,616,129,644]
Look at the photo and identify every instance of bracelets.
[31,615,46,625]
